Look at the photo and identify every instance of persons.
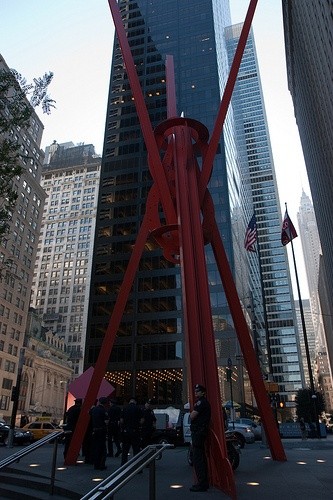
[60,396,158,475]
[188,383,212,491]
[299,417,316,440]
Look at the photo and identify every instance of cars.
[0,424,33,447]
[152,409,254,446]
[21,419,65,443]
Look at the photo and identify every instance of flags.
[243,214,260,254]
[280,210,299,246]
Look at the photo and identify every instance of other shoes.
[190,483,210,492]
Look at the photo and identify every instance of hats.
[194,384,206,392]
